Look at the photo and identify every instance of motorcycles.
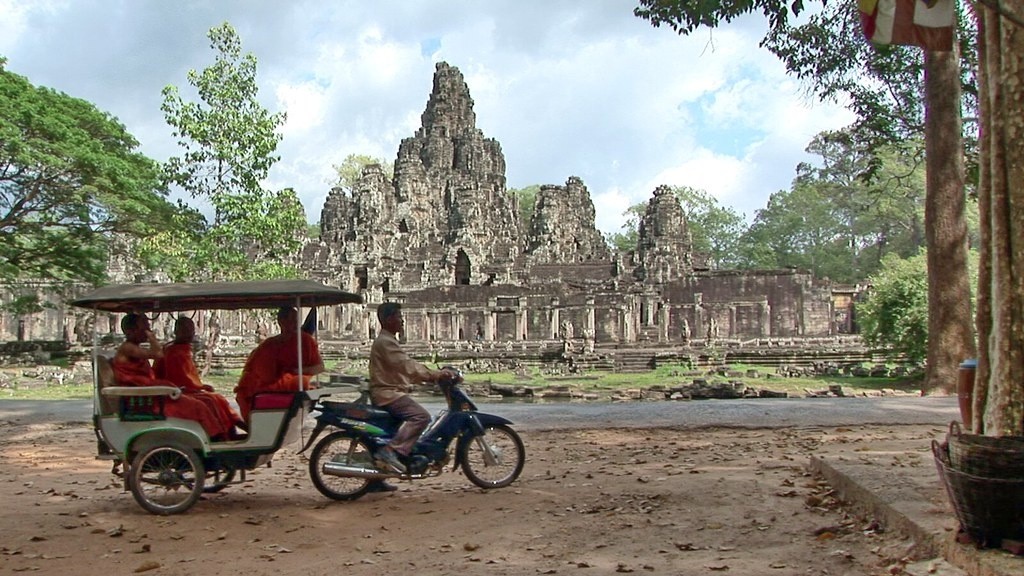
[298,359,525,503]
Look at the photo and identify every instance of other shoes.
[372,447,408,472]
[365,480,398,492]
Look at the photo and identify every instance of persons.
[153,317,248,439]
[370,303,459,460]
[112,313,228,441]
[235,308,325,422]
